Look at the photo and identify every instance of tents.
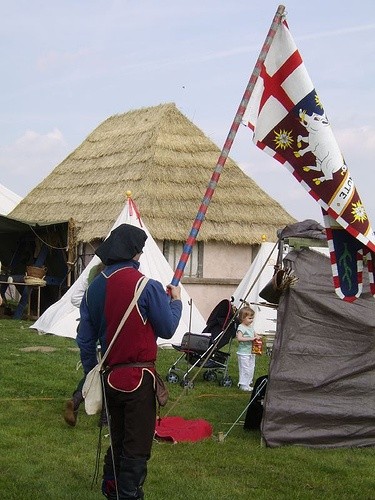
[0,184,375,451]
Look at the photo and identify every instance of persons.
[236,307,262,392]
[64,262,106,427]
[75,224,182,500]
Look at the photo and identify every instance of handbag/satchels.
[82,362,103,415]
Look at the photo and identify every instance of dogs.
[297,109,348,185]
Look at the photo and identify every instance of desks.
[0,281,41,321]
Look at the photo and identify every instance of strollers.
[167,296,250,390]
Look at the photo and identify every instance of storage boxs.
[24,276,47,287]
[27,266,47,278]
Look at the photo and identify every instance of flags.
[241,11,375,302]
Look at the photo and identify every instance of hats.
[94,223,148,266]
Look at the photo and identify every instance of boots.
[64,390,111,426]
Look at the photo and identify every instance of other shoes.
[238,385,254,392]
